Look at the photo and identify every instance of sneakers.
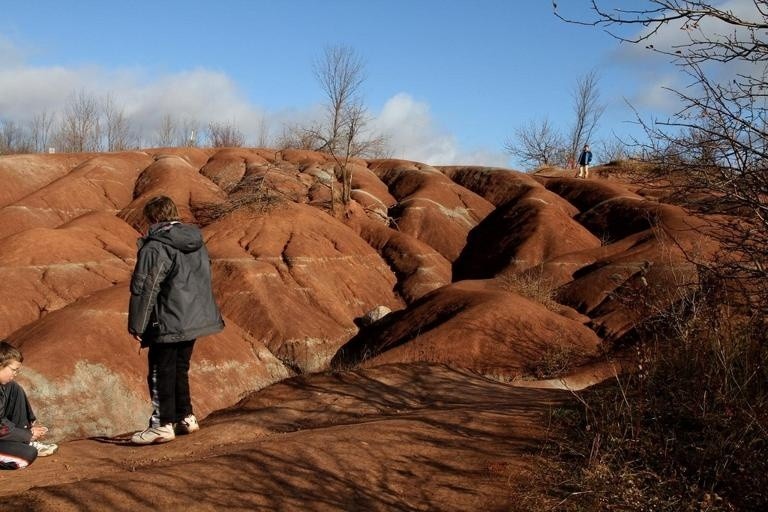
[174,413,200,435]
[29,438,59,457]
[131,421,176,444]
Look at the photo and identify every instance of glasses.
[6,364,21,375]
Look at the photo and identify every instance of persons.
[574,145,593,180]
[0,343,59,471]
[123,195,224,448]
